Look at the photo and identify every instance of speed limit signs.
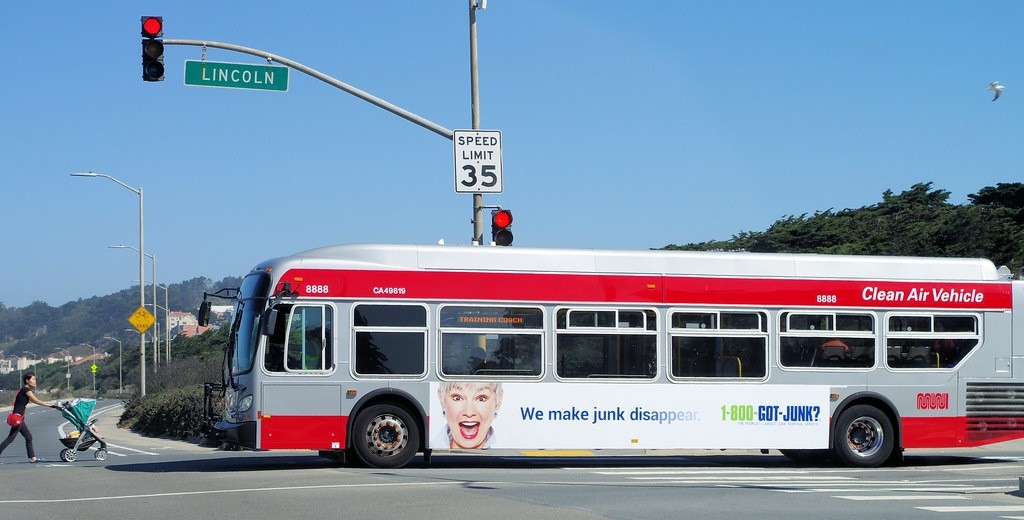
[455,129,504,194]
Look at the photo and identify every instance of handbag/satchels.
[7,414,23,428]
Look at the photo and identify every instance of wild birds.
[985,79,1007,102]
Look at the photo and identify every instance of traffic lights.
[140,14,168,82]
[492,210,516,246]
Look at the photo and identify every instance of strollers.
[52,395,110,464]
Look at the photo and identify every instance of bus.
[197,242,1022,468]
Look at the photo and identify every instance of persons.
[0,373,56,463]
[460,347,488,375]
[434,382,504,450]
[819,337,849,352]
[932,320,955,349]
[275,323,321,369]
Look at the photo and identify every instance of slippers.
[30,458,44,462]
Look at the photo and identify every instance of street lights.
[12,278,172,397]
[70,170,147,401]
[108,244,158,376]
